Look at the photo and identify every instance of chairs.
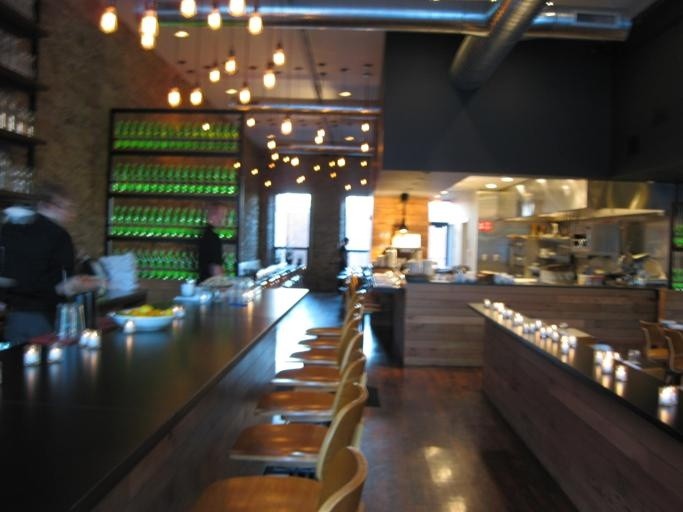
[226,273,383,479]
[638,320,681,383]
[196,445,368,509]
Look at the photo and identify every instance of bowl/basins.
[105,305,186,332]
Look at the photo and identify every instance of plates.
[376,249,399,267]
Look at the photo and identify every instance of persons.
[338,238,350,269]
[1,173,108,346]
[195,200,227,286]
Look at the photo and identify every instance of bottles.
[110,117,239,283]
[55,301,87,342]
[0,97,38,199]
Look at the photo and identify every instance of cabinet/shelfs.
[1,1,47,206]
[503,216,536,242]
[104,107,243,278]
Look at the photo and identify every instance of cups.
[179,283,195,297]
